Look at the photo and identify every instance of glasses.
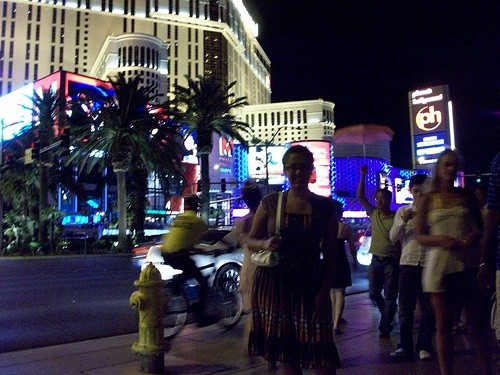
[287,165,311,171]
[375,197,388,201]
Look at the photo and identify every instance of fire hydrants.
[129,261,173,375]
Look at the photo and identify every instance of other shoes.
[379,325,393,338]
[198,318,218,326]
[174,277,182,296]
[332,318,346,325]
[333,329,341,335]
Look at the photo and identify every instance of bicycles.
[162,248,244,341]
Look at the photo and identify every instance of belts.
[373,255,394,261]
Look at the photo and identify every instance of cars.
[141,229,244,296]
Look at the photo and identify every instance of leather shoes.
[390,347,414,359]
[418,350,432,359]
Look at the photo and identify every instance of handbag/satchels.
[445,271,470,290]
[250,192,284,267]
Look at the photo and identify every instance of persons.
[160,144,500,374]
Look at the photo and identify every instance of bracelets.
[258,239,263,249]
[462,238,467,246]
